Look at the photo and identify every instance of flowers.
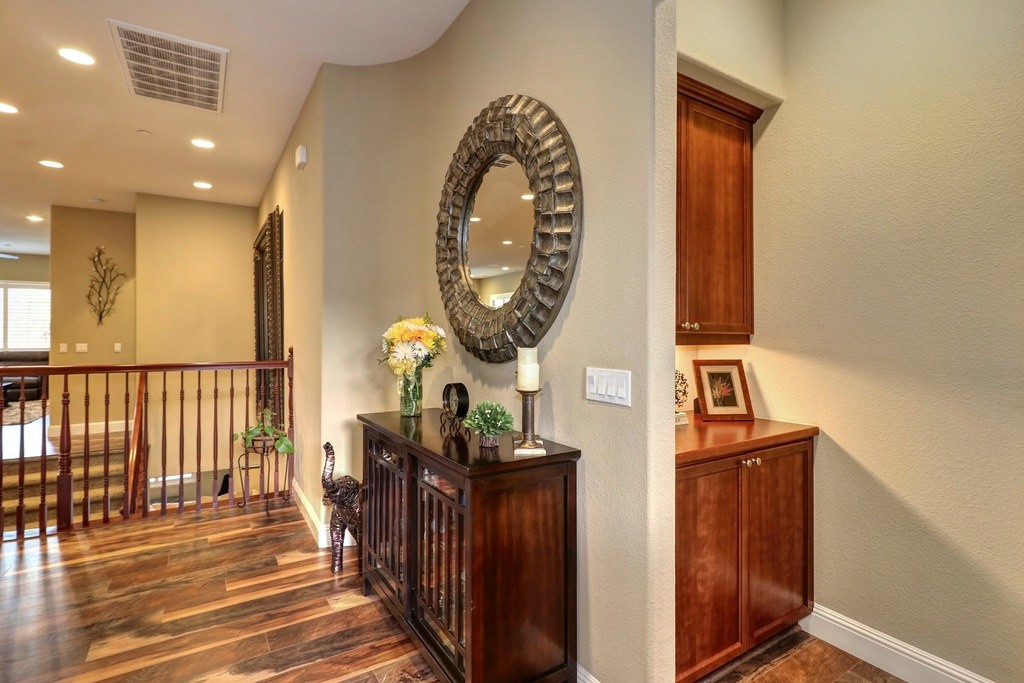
[376,309,448,376]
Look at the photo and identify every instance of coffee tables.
[1,382,15,407]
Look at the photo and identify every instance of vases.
[400,368,422,417]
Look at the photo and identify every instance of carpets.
[3,399,49,426]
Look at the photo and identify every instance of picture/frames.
[253,204,285,432]
[692,359,756,421]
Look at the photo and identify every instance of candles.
[517,346,537,363]
[518,357,540,391]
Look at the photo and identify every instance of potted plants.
[233,399,296,453]
[462,400,514,447]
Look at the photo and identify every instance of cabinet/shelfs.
[357,407,581,683]
[675,73,765,345]
[674,410,818,683]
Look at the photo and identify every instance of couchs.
[0,350,49,401]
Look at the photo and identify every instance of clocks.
[442,383,469,418]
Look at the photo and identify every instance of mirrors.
[435,94,583,365]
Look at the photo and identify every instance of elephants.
[320,441,364,575]
